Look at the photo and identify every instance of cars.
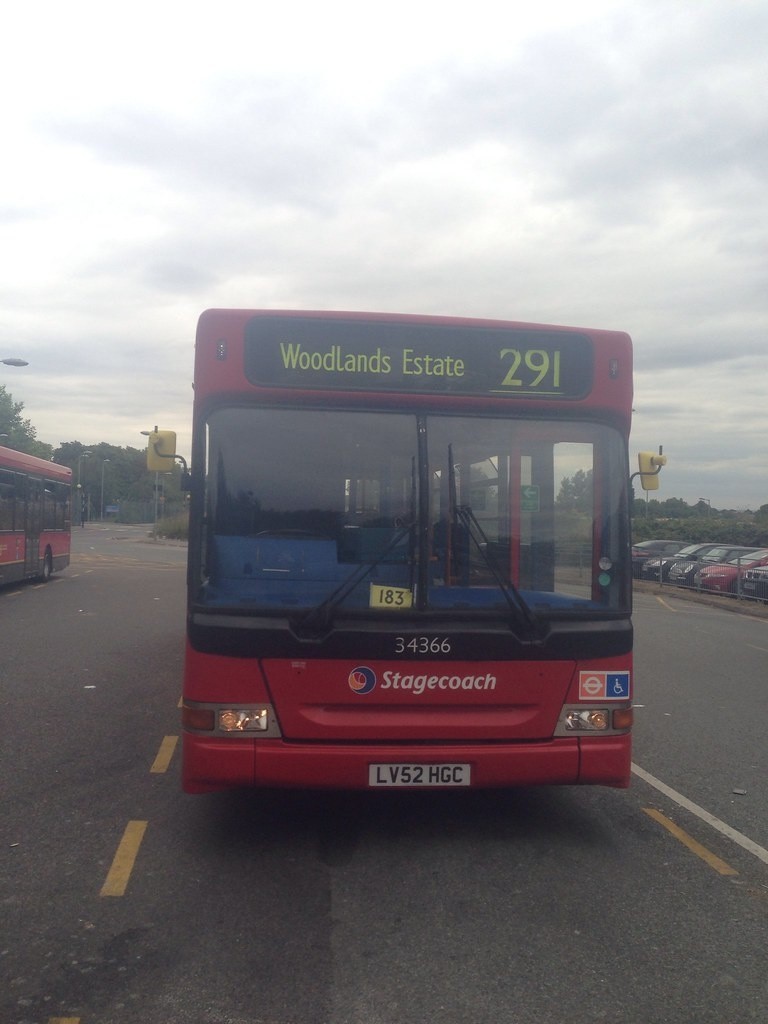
[741,565,768,605]
[669,546,766,585]
[631,540,692,579]
[641,542,734,580]
[694,548,768,596]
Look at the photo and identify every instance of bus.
[468,510,532,583]
[143,307,668,794]
[0,446,73,585]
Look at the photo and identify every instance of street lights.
[141,431,158,536]
[700,497,711,516]
[101,458,111,519]
[161,470,173,520]
[77,450,92,525]
